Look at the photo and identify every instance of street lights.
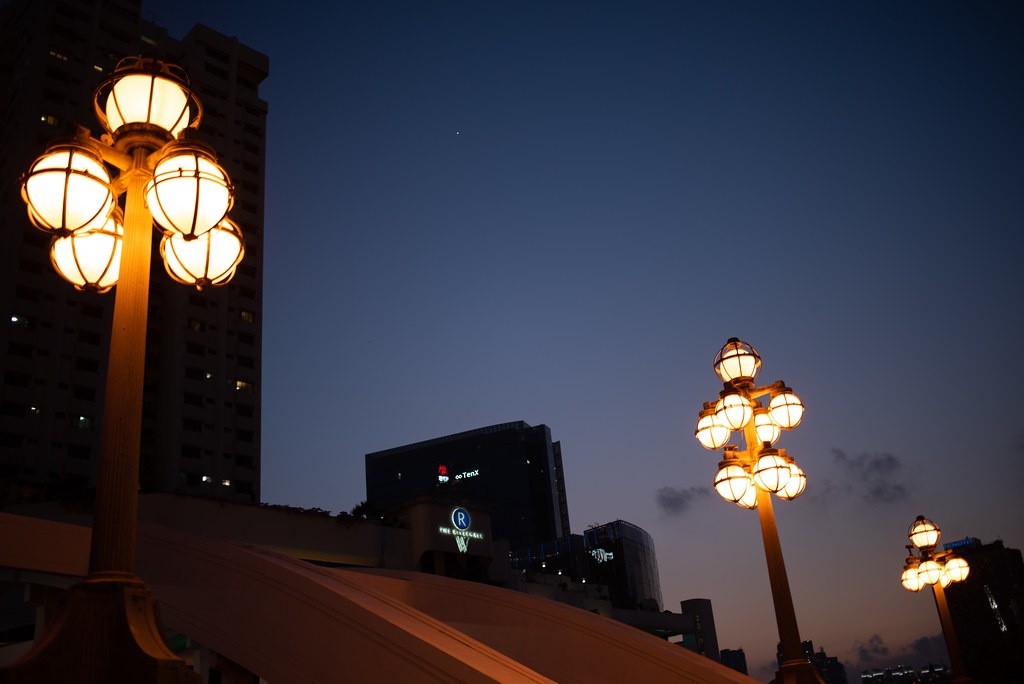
[1,67,244,684]
[691,336,825,684]
[899,514,972,684]
